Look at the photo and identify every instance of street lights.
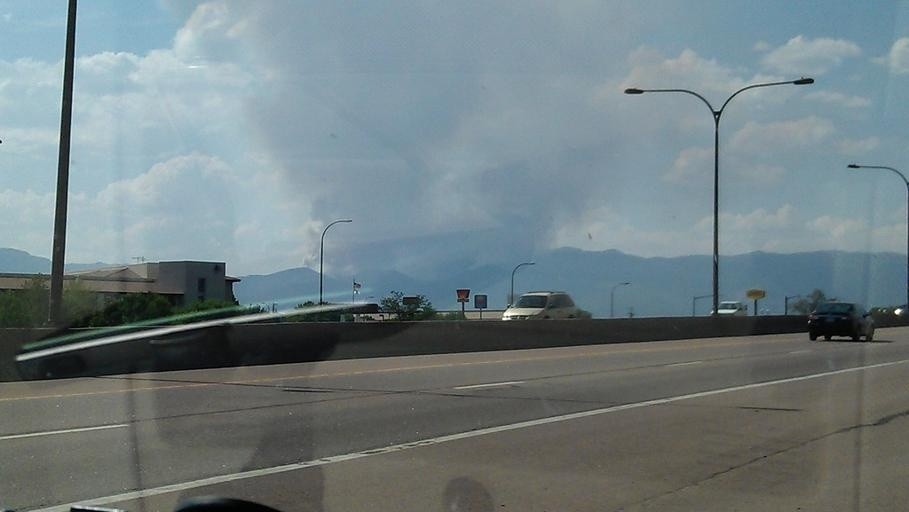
[609,280,632,318]
[845,160,908,312]
[272,301,278,312]
[782,292,804,315]
[350,274,361,301]
[620,72,819,315]
[316,217,353,304]
[378,314,384,320]
[509,260,535,307]
[692,293,723,317]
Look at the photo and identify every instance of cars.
[869,304,908,318]
[805,298,876,343]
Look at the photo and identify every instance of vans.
[709,301,748,316]
[499,287,576,321]
